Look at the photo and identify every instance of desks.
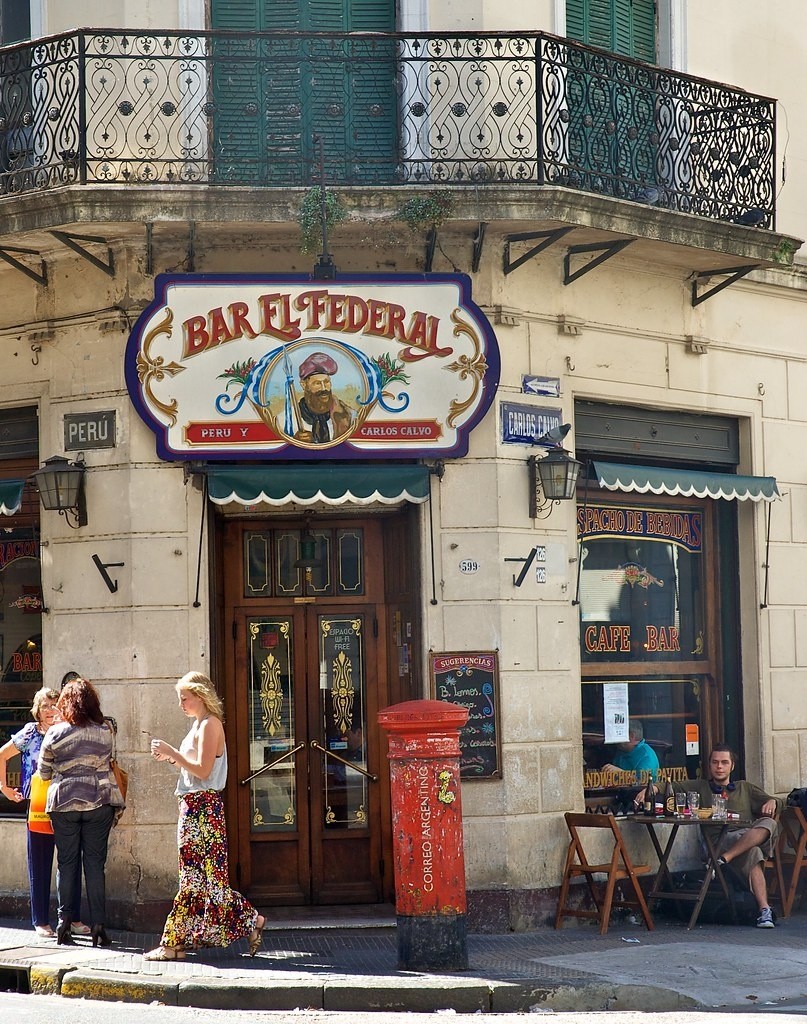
[628,815,750,930]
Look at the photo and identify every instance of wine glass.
[676,793,686,818]
[712,794,725,819]
[687,792,700,819]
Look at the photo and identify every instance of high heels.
[71,922,91,934]
[56,916,77,945]
[33,924,54,937]
[90,924,112,947]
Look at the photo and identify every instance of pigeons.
[534,423,571,448]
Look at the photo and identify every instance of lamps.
[528,444,585,521]
[312,259,337,282]
[7,594,43,608]
[736,209,765,227]
[29,450,90,528]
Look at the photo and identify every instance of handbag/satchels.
[28,771,57,834]
[103,719,128,818]
[786,787,807,808]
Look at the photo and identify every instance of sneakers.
[756,908,774,928]
[702,856,724,879]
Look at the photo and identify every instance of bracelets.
[169,761,176,764]
[168,757,171,760]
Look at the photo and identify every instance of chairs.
[771,806,807,915]
[554,813,654,933]
[714,808,789,919]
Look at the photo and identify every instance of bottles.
[644,777,655,815]
[664,775,674,816]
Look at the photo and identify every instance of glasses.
[39,703,57,712]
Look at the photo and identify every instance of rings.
[769,810,771,812]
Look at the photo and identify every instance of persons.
[601,720,660,784]
[0,687,91,937]
[38,678,126,947]
[143,671,268,961]
[637,743,783,928]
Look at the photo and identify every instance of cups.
[588,769,599,783]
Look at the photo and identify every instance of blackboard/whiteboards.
[428,648,505,782]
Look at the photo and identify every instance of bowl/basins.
[698,810,712,818]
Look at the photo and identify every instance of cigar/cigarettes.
[634,800,639,805]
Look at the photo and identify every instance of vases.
[60,151,81,169]
[552,175,579,186]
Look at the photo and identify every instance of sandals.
[248,916,267,957]
[143,945,186,961]
